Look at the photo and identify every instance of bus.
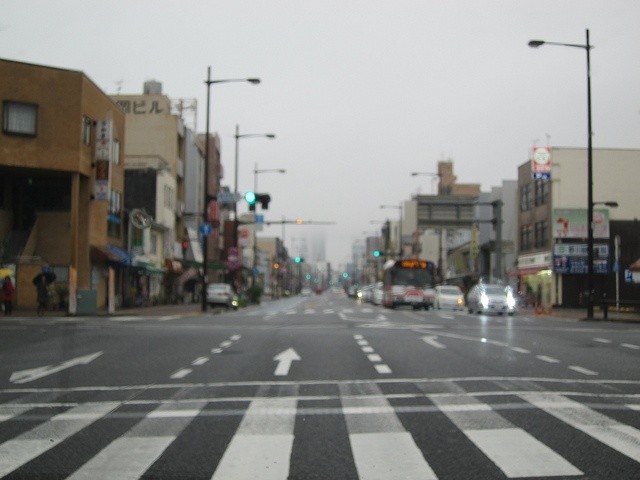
[383,259,434,311]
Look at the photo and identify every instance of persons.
[37,276,51,315]
[0,275,15,316]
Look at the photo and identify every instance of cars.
[359,282,382,304]
[466,284,515,317]
[433,286,465,310]
[206,283,239,309]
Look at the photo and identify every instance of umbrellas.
[33,270,56,287]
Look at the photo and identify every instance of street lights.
[203,67,261,309]
[233,124,276,291]
[411,172,445,278]
[529,30,594,320]
[585,201,620,321]
[254,164,286,269]
